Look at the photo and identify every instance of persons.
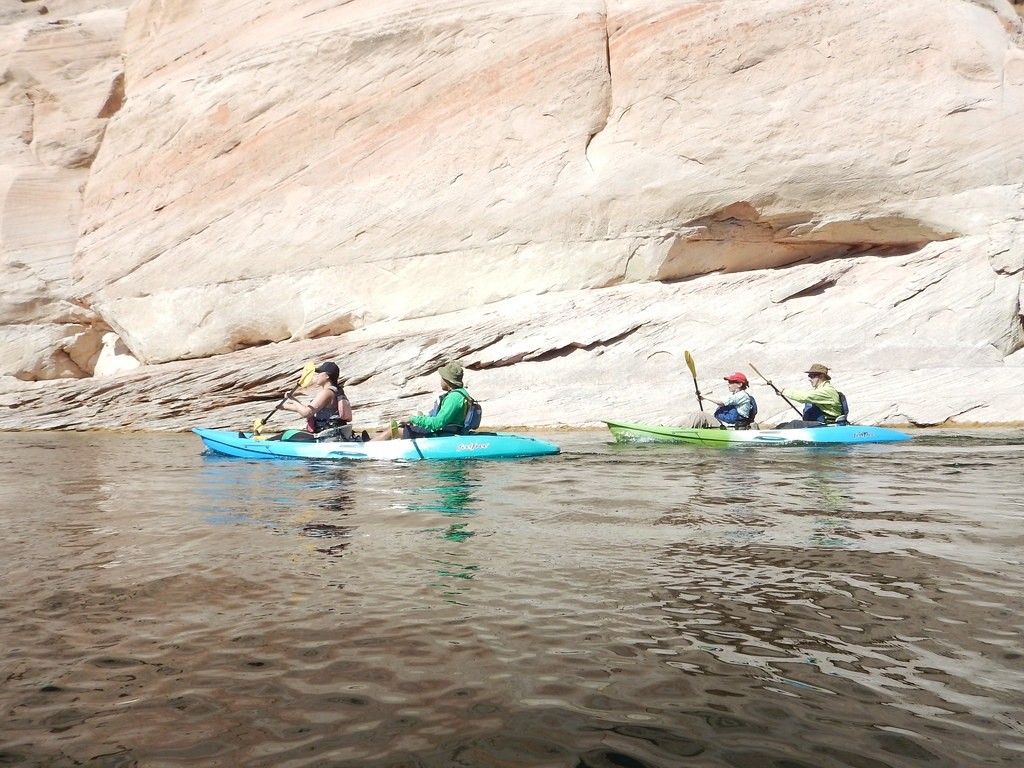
[238,361,344,444]
[363,360,471,442]
[750,363,843,430]
[661,371,752,430]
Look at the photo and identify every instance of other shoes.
[361,429,370,442]
[238,431,247,439]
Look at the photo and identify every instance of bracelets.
[782,389,785,393]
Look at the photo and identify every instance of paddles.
[390,420,405,439]
[748,361,802,418]
[252,362,316,437]
[683,350,703,413]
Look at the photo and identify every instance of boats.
[190,423,566,462]
[597,418,916,453]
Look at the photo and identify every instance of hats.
[438,363,463,388]
[315,360,339,375]
[724,371,748,385]
[804,364,832,380]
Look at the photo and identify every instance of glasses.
[808,373,821,379]
